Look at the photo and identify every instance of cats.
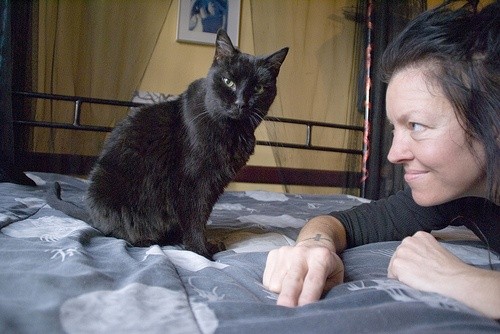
[83,27,290,262]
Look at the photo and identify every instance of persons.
[261,0,500,317]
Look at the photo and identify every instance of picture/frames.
[176,0,243,49]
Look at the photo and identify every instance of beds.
[0,89,500,334]
